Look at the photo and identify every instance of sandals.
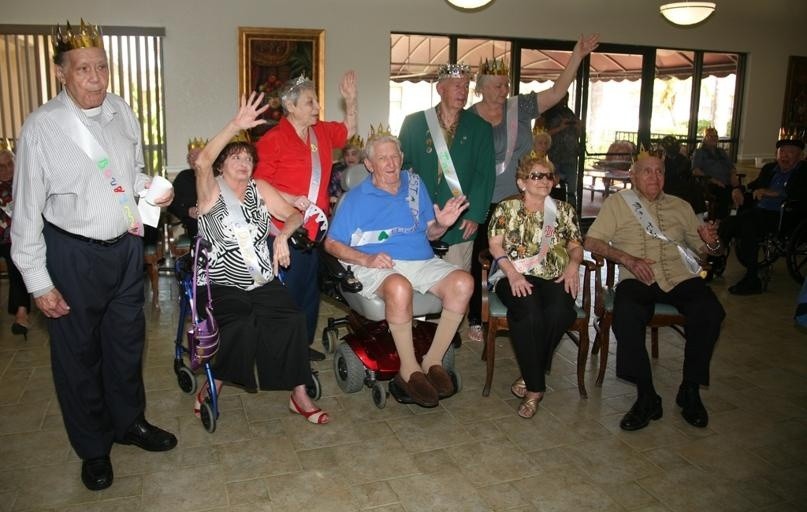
[288,396,329,424]
[510,376,529,397]
[194,380,222,416]
[517,393,543,417]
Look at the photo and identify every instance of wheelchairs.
[712,182,807,292]
[174,239,321,434]
[323,241,462,410]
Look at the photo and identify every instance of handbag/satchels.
[189,309,222,370]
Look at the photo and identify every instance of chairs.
[596,140,635,188]
[705,173,745,216]
[590,250,716,389]
[688,175,715,212]
[477,250,595,398]
[168,223,192,254]
[143,216,166,311]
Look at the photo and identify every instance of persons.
[0,135,31,341]
[323,123,474,408]
[252,72,358,361]
[583,140,726,431]
[487,151,584,419]
[397,62,497,348]
[10,18,178,490]
[167,137,210,247]
[655,127,807,295]
[194,89,329,424]
[466,33,599,341]
[532,126,552,158]
[328,135,364,198]
[535,92,581,208]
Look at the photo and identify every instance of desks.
[583,168,630,201]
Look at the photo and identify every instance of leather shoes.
[420,361,453,397]
[728,277,761,295]
[675,385,708,427]
[306,345,328,362]
[80,440,112,490]
[620,395,662,430]
[395,369,438,408]
[113,420,177,451]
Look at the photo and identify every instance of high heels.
[10,320,32,342]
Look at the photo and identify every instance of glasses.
[526,172,554,181]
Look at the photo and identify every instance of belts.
[50,222,130,247]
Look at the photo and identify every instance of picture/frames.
[781,56,807,143]
[237,25,325,126]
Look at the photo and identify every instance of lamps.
[659,0,717,26]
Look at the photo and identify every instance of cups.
[144,176,173,206]
